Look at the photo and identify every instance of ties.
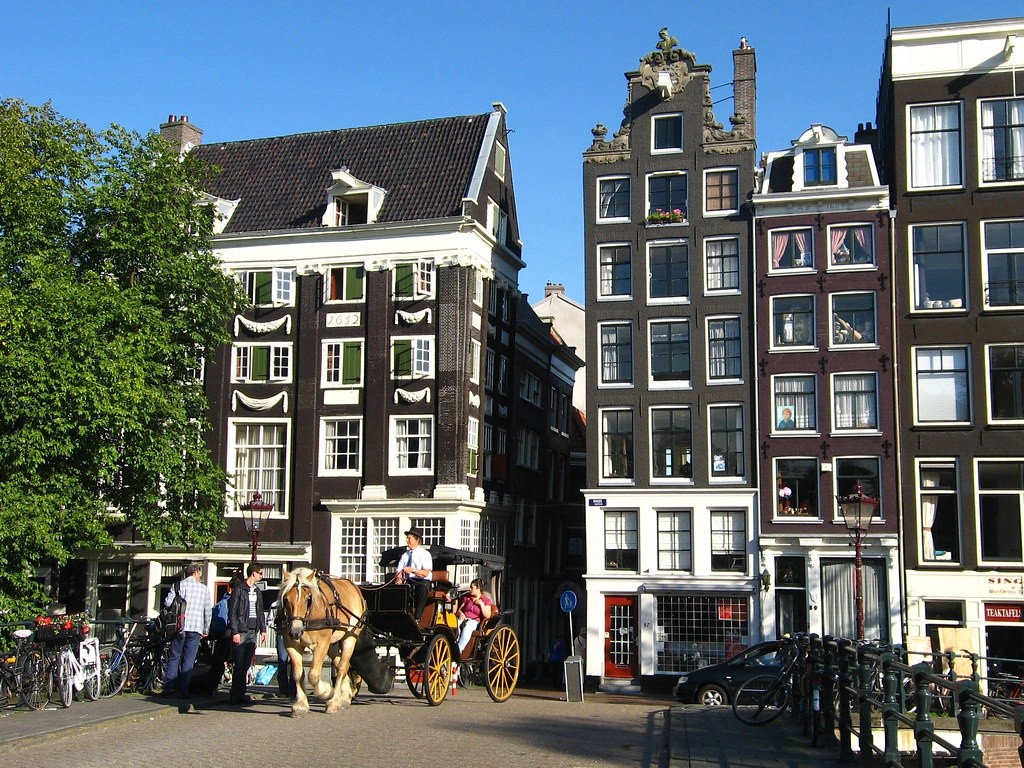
[405,552,412,577]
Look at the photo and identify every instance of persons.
[455,578,493,655]
[210,562,298,706]
[394,525,433,624]
[163,564,213,701]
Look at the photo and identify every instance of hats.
[580,628,587,634]
[405,527,423,539]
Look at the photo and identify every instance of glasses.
[257,571,262,575]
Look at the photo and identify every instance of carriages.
[274,544,522,721]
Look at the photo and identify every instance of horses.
[278,566,368,720]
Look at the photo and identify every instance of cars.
[659,629,747,669]
[672,640,792,707]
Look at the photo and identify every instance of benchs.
[386,571,450,629]
[452,600,499,660]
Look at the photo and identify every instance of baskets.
[36,627,85,644]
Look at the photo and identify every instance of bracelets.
[411,568,416,574]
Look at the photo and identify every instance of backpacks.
[212,593,231,632]
[155,581,186,641]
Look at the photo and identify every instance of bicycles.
[0,608,213,711]
[733,629,1024,725]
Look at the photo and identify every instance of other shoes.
[229,694,249,705]
[415,619,421,626]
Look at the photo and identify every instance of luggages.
[192,635,227,696]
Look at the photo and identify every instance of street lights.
[836,484,881,641]
[237,490,277,563]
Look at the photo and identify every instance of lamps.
[760,568,771,592]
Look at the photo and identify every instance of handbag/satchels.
[256,664,278,686]
